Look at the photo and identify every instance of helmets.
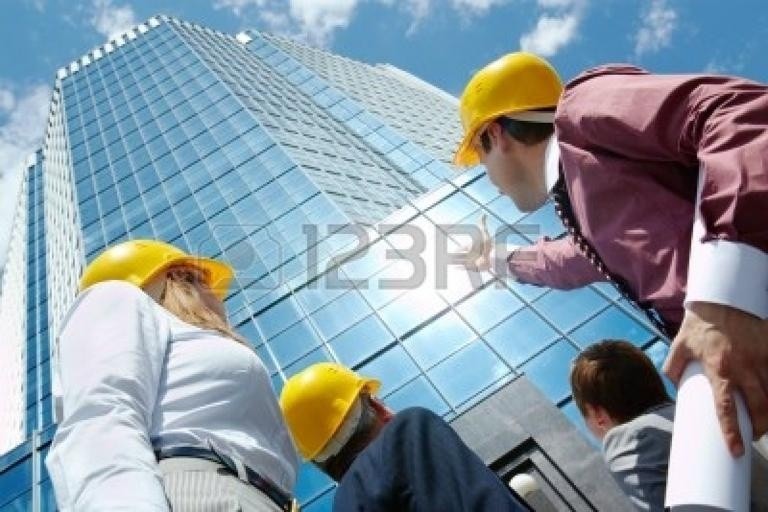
[78,239,235,301]
[455,52,564,167]
[280,362,381,462]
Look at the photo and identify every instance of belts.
[155,447,297,512]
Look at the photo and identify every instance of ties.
[552,163,672,340]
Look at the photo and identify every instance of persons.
[43,52,768,512]
